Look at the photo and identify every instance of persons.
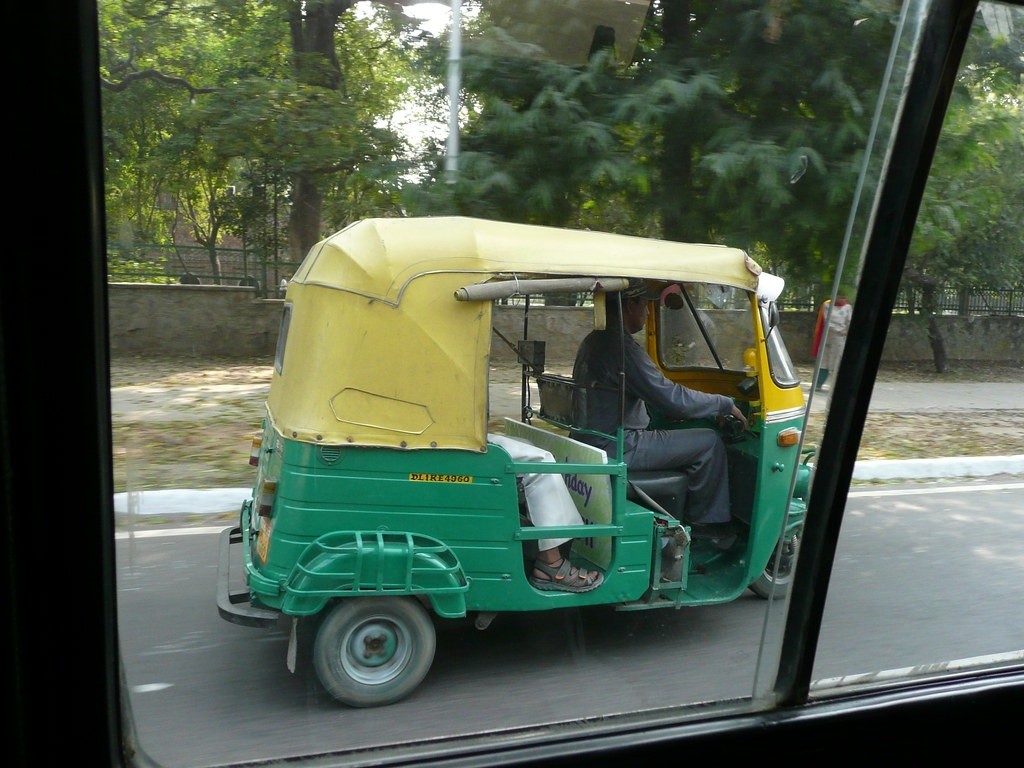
[280,275,287,290]
[487,432,604,593]
[811,294,852,392]
[569,297,750,539]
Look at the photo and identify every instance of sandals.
[529,556,604,592]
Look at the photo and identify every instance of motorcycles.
[215,215,826,709]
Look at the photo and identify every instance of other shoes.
[685,520,745,538]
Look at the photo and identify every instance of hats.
[605,278,660,301]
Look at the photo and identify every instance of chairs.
[539,373,687,522]
